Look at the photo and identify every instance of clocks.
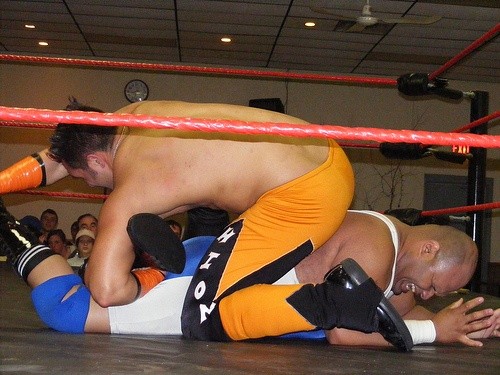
[124,79,149,103]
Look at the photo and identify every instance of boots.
[0,196,43,272]
[126,213,186,274]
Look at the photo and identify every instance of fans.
[309,0,441,33]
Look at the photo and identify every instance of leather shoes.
[323,257,414,352]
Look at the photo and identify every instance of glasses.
[78,239,94,244]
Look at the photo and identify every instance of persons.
[0,95,414,355]
[0,208,100,268]
[0,193,500,347]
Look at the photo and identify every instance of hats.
[75,229,96,240]
